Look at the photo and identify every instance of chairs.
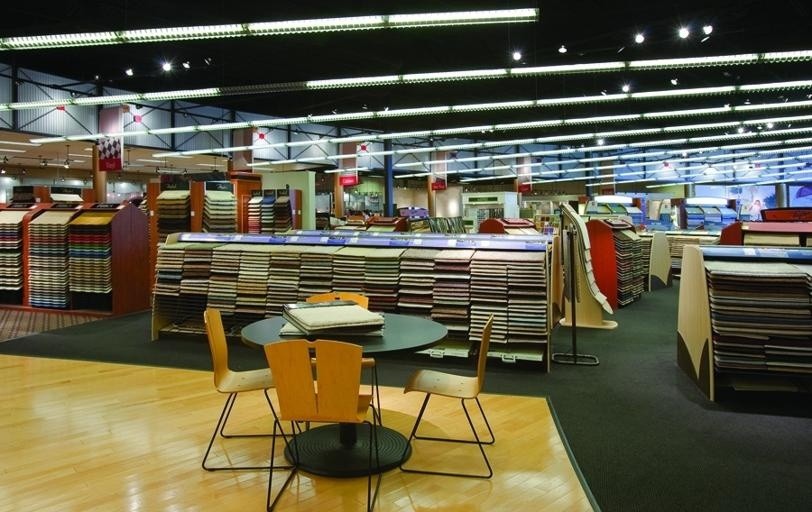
[399,315,495,478]
[264,339,382,512]
[305,293,382,430]
[202,309,298,471]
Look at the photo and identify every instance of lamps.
[0,157,6,174]
[383,105,389,111]
[63,146,70,169]
[308,113,312,118]
[728,70,743,84]
[724,95,736,108]
[429,136,434,142]
[183,61,191,69]
[601,89,608,96]
[39,157,48,169]
[205,57,212,65]
[69,89,77,96]
[664,73,681,89]
[362,104,368,110]
[778,95,787,101]
[744,96,752,104]
[332,109,338,114]
[552,40,568,57]
[696,23,714,48]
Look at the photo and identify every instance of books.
[277,300,386,338]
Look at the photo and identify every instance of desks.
[241,314,448,478]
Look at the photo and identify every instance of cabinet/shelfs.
[0,203,151,318]
[586,221,644,310]
[671,198,737,229]
[466,201,505,229]
[151,233,560,374]
[334,216,409,232]
[242,172,314,233]
[640,234,672,292]
[151,175,260,299]
[720,220,812,245]
[677,244,812,417]
[479,218,536,234]
[581,200,629,220]
